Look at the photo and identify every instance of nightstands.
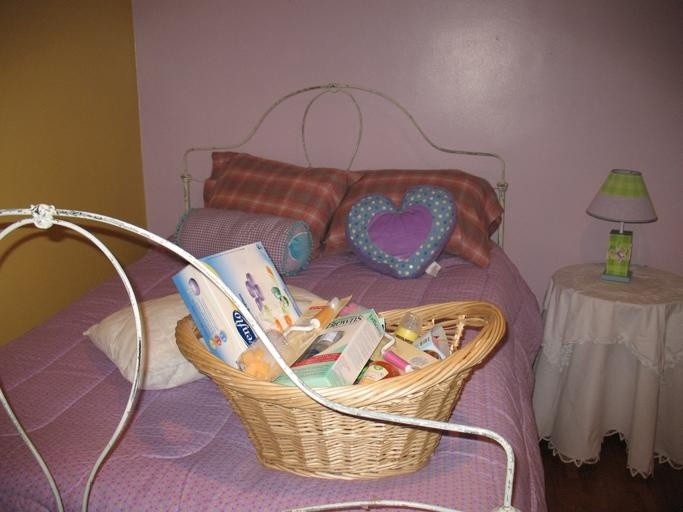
[527,261,682,479]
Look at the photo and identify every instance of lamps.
[584,167,661,283]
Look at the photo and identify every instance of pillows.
[168,203,315,277]
[77,274,324,394]
[343,183,459,282]
[321,161,504,268]
[197,146,351,256]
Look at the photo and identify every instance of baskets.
[175,282,509,482]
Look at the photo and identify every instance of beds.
[1,80,552,511]
